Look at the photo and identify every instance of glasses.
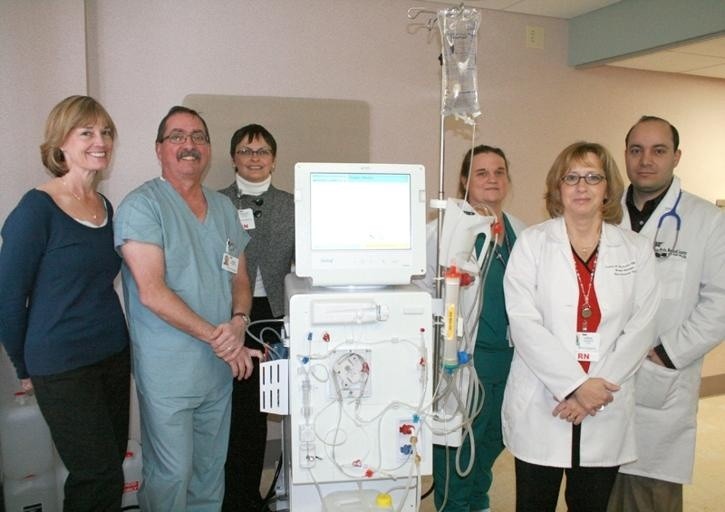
[561,173,607,185]
[160,133,210,145]
[234,147,273,158]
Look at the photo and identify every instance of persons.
[427,144,527,512]
[605,115,725,512]
[501,140,662,512]
[112,104,262,512]
[215,123,295,512]
[0,94,132,511]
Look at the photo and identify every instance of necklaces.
[56,176,98,219]
[568,228,601,320]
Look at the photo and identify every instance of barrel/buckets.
[0,392,143,512]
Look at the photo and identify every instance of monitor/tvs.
[295,162,427,289]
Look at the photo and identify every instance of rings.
[596,404,605,413]
[226,347,234,352]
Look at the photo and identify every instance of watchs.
[231,312,250,326]
